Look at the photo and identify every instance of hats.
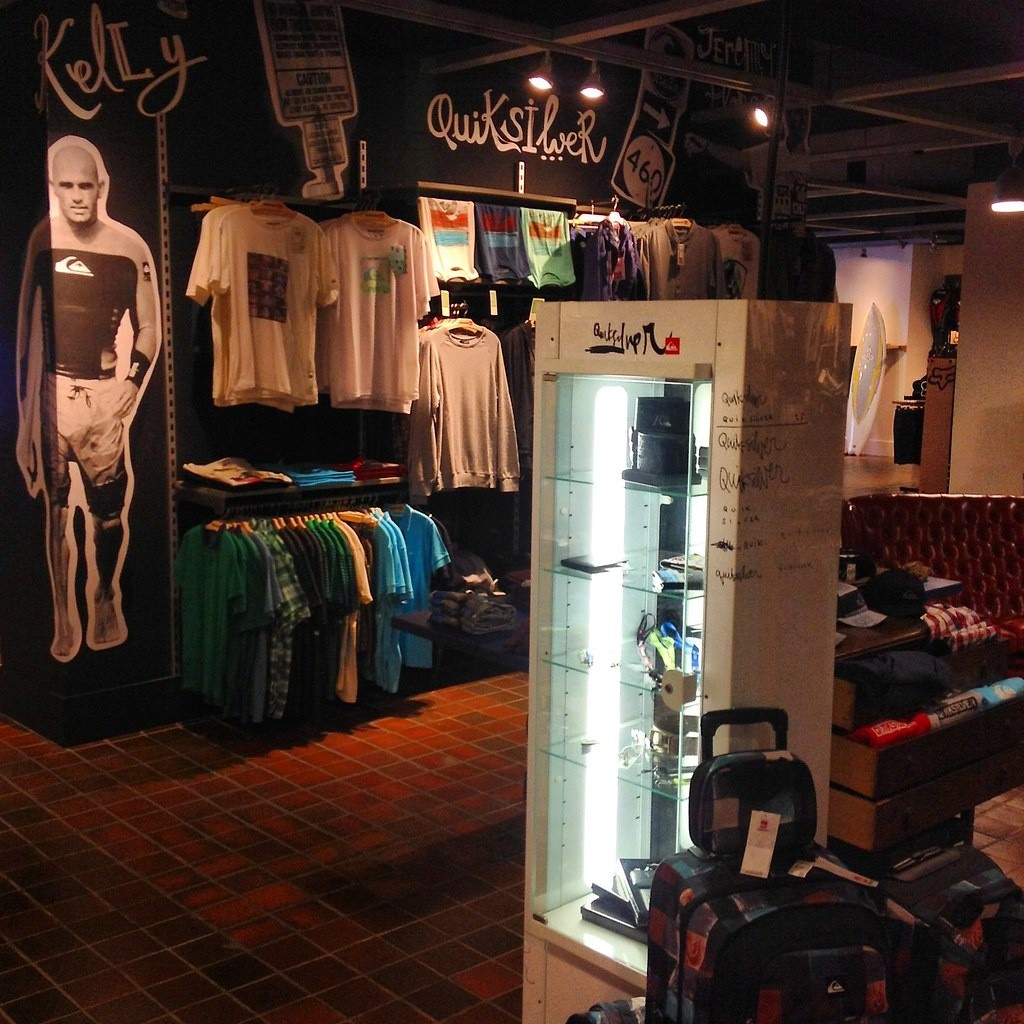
[837,582,888,627]
[863,568,927,617]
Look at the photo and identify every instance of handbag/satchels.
[565,996,646,1024]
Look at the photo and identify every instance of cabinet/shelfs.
[830,574,1024,844]
[163,182,408,503]
[543,466,707,802]
[420,180,624,298]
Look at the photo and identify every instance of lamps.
[754,102,776,129]
[895,237,908,250]
[859,248,866,257]
[991,139,1024,213]
[579,58,605,99]
[928,238,938,252]
[528,53,554,89]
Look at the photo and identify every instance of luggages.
[875,817,1024,1024]
[645,706,890,1024]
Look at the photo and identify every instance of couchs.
[841,493,1024,659]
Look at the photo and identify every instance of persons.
[19,145,157,654]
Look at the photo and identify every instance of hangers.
[648,204,691,231]
[718,215,743,235]
[433,300,488,336]
[767,212,807,239]
[892,400,927,410]
[191,182,295,220]
[345,190,397,231]
[203,492,408,535]
[565,196,624,233]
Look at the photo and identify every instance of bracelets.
[123,348,151,387]
